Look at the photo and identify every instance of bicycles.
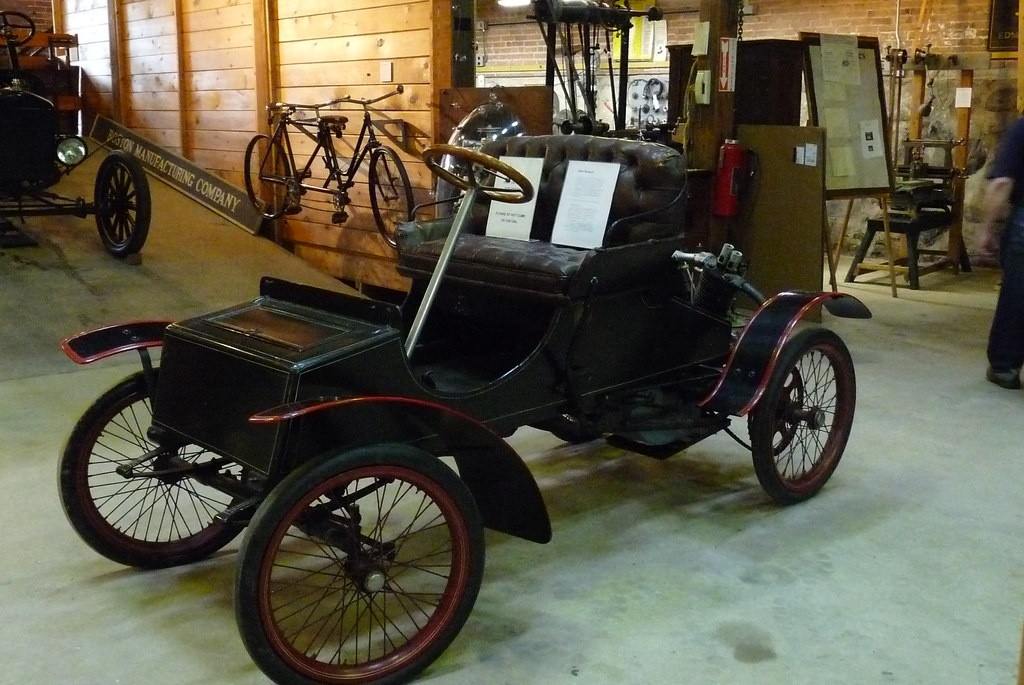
[244,84,416,248]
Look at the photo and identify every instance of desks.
[845,211,972,290]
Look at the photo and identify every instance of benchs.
[394,135,685,305]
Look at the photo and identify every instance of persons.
[977,112,1023,391]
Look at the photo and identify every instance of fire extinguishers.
[713,135,745,217]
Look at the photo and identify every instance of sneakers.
[986,366,1021,389]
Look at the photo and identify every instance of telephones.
[695,70,711,105]
